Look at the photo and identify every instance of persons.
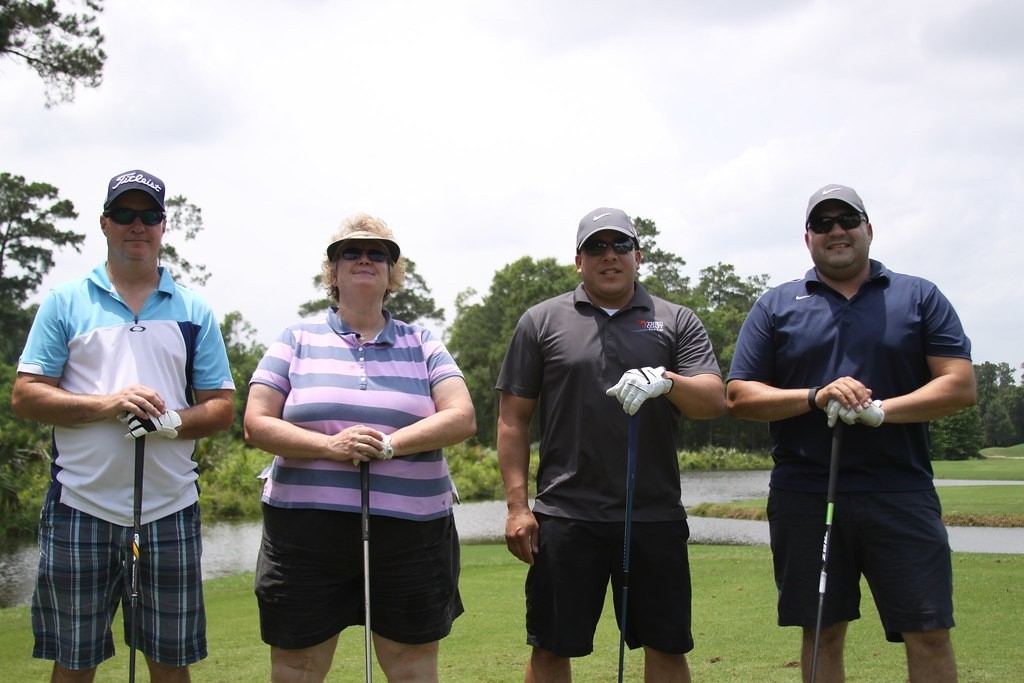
[494,208,727,683]
[11,168,237,683]
[726,183,976,683]
[243,214,476,683]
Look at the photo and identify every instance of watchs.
[808,385,827,413]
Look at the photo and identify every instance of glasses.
[806,211,868,233]
[335,247,392,267]
[103,206,167,226]
[582,234,637,256]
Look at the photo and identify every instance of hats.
[326,230,401,266]
[102,170,166,211]
[576,207,640,256]
[804,183,870,233]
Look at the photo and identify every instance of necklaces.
[357,314,386,341]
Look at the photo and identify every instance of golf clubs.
[124,415,144,683]
[354,461,375,683]
[615,403,637,682]
[809,418,840,683]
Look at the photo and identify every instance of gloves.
[606,365,674,417]
[116,401,183,440]
[352,427,395,466]
[822,392,884,429]
